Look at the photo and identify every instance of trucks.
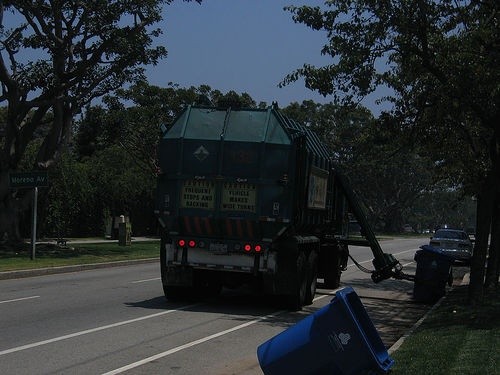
[158,101,350,312]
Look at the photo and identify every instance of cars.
[430,229,475,262]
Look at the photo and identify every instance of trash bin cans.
[257,284,395,375]
[412,245,456,306]
